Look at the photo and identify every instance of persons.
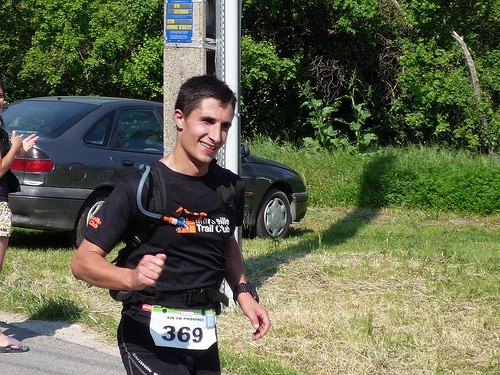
[68,73,272,375]
[0,84,41,279]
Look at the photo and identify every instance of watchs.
[231,281,260,305]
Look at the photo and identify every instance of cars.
[1,96,309,243]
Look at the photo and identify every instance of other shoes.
[0,328,29,352]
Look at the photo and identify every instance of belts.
[134,287,229,307]
